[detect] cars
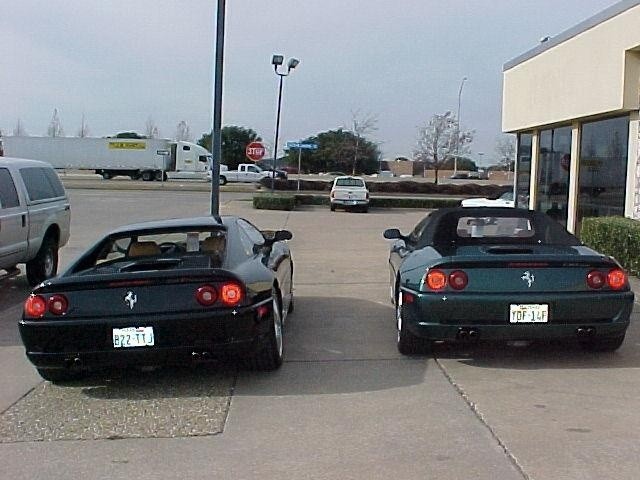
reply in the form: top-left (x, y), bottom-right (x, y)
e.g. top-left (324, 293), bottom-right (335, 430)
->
top-left (320, 170), bottom-right (490, 180)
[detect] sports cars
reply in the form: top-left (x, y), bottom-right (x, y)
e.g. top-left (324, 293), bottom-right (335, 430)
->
top-left (381, 206), bottom-right (635, 358)
top-left (16, 213), bottom-right (295, 387)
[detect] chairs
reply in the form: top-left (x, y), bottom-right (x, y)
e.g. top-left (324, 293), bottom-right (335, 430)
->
top-left (201, 237), bottom-right (227, 256)
top-left (128, 240), bottom-right (161, 257)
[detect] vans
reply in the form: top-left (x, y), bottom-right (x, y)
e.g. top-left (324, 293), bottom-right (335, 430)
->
top-left (0, 156), bottom-right (72, 289)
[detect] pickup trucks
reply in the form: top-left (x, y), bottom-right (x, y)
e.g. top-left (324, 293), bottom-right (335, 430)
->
top-left (218, 163), bottom-right (273, 185)
top-left (328, 175), bottom-right (371, 213)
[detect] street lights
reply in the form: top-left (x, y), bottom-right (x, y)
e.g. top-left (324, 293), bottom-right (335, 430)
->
top-left (270, 53), bottom-right (300, 193)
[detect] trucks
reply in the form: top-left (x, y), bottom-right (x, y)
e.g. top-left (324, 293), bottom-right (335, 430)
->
top-left (0, 135), bottom-right (213, 182)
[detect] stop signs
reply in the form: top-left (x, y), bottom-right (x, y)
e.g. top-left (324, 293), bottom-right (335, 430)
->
top-left (245, 141), bottom-right (266, 162)
